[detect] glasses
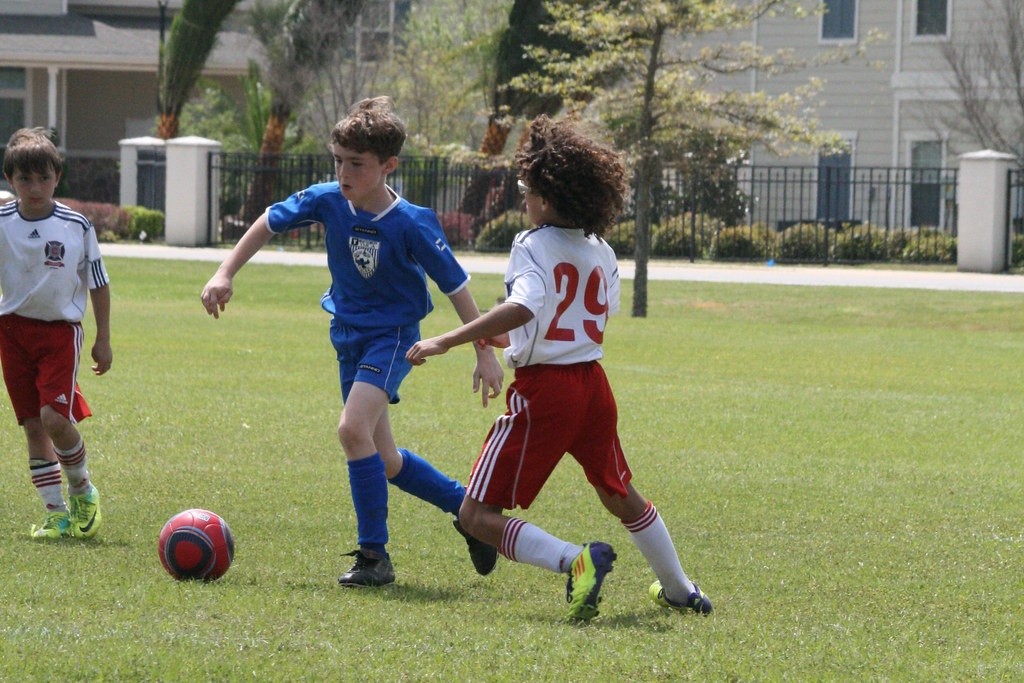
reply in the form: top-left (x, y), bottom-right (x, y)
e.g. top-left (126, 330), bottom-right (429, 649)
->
top-left (517, 180), bottom-right (537, 194)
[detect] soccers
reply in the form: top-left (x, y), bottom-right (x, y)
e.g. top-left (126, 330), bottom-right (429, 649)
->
top-left (158, 509), bottom-right (235, 583)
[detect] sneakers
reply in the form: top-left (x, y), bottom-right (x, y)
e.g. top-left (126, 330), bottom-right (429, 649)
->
top-left (566, 541), bottom-right (618, 625)
top-left (453, 487), bottom-right (503, 576)
top-left (649, 581), bottom-right (711, 614)
top-left (338, 550), bottom-right (396, 587)
top-left (33, 513), bottom-right (70, 539)
top-left (69, 484), bottom-right (101, 540)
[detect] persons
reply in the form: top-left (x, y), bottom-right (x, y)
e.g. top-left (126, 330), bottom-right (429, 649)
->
top-left (200, 94), bottom-right (506, 589)
top-left (405, 119), bottom-right (713, 625)
top-left (0, 126), bottom-right (113, 538)
